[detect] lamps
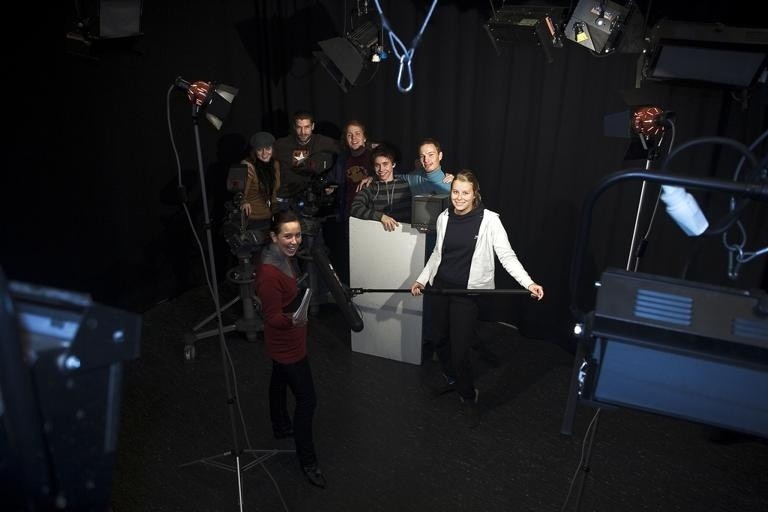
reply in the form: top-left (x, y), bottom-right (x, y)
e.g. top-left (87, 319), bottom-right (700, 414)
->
top-left (166, 75), bottom-right (310, 511)
top-left (563, 107), bottom-right (675, 510)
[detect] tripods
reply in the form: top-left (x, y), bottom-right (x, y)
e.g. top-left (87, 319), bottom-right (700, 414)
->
top-left (174, 114), bottom-right (298, 511)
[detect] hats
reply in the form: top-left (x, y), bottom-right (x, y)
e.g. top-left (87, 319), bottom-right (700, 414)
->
top-left (251, 132), bottom-right (275, 148)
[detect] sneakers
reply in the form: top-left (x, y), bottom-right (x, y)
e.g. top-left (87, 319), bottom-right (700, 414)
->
top-left (457, 389), bottom-right (480, 428)
top-left (275, 429), bottom-right (293, 438)
top-left (304, 469), bottom-right (328, 489)
top-left (418, 373), bottom-right (455, 397)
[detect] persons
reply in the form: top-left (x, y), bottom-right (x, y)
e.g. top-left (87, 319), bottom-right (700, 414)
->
top-left (223, 117), bottom-right (454, 341)
top-left (411, 170), bottom-right (543, 424)
top-left (256, 210), bottom-right (326, 487)
